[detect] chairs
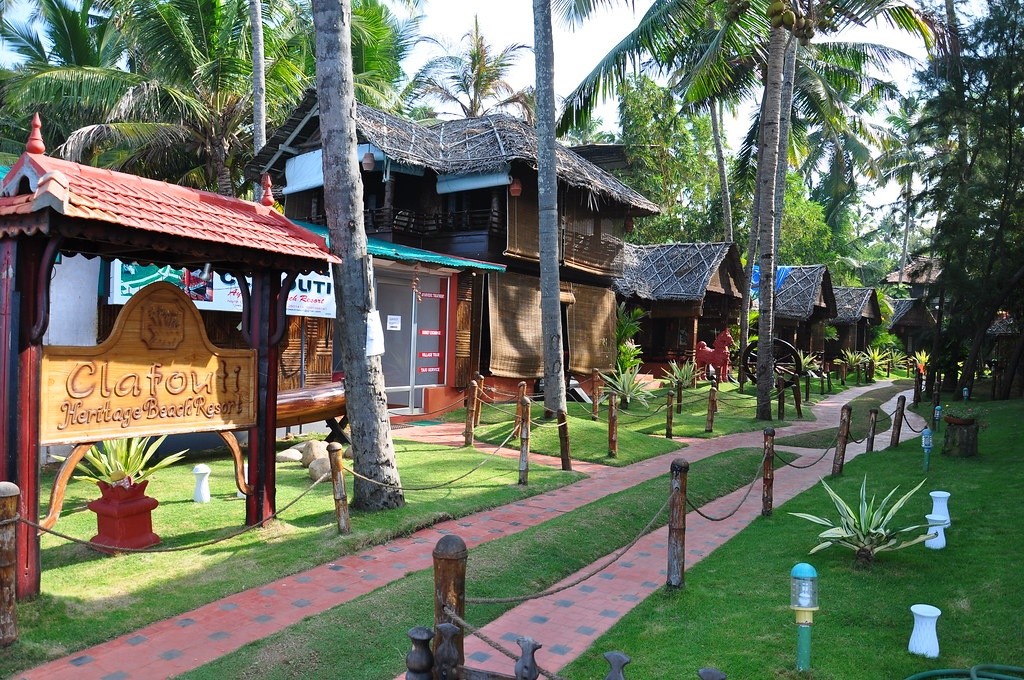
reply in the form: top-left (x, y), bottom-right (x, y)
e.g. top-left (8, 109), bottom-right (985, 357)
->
top-left (394, 210), bottom-right (415, 232)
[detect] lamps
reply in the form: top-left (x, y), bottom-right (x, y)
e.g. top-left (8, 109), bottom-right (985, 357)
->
top-left (791, 563), bottom-right (820, 611)
top-left (963, 388), bottom-right (969, 397)
top-left (934, 406), bottom-right (942, 418)
top-left (510, 176), bottom-right (522, 196)
top-left (921, 428), bottom-right (934, 448)
top-left (362, 140), bottom-right (375, 171)
top-left (624, 214), bottom-right (634, 232)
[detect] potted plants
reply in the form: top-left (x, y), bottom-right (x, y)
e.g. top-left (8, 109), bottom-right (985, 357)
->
top-left (942, 407), bottom-right (990, 425)
top-left (49, 434), bottom-right (190, 554)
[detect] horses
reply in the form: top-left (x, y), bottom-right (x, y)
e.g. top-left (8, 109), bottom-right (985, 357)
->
top-left (696, 327), bottom-right (734, 383)
